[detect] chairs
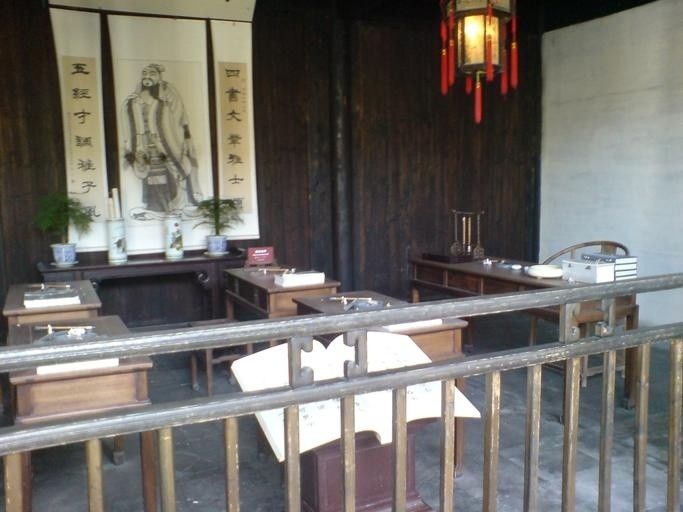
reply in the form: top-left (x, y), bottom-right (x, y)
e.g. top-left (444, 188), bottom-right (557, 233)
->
top-left (528, 241), bottom-right (632, 389)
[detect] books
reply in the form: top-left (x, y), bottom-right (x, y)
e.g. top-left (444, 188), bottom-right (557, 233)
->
top-left (273, 269), bottom-right (327, 289)
top-left (23, 289), bottom-right (80, 309)
top-left (582, 252), bottom-right (637, 281)
top-left (384, 319), bottom-right (443, 330)
top-left (37, 338), bottom-right (120, 376)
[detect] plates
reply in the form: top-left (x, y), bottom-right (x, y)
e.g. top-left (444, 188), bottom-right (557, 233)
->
top-left (527, 264), bottom-right (564, 278)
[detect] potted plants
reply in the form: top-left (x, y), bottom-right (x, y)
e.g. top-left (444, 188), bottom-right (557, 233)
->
top-left (195, 198), bottom-right (244, 256)
top-left (32, 194), bottom-right (95, 268)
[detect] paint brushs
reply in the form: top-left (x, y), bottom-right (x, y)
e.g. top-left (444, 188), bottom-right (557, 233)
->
top-left (29, 285), bottom-right (73, 288)
top-left (36, 325), bottom-right (96, 330)
top-left (328, 297), bottom-right (372, 300)
top-left (258, 268), bottom-right (297, 271)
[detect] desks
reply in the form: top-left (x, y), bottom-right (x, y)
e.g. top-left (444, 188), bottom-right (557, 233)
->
top-left (225, 267), bottom-right (342, 351)
top-left (7, 315), bottom-right (156, 511)
top-left (3, 281), bottom-right (102, 330)
top-left (292, 289), bottom-right (469, 479)
top-left (409, 257), bottom-right (640, 409)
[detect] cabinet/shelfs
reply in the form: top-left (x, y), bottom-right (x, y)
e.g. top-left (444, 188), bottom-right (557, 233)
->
top-left (36, 248), bottom-right (248, 320)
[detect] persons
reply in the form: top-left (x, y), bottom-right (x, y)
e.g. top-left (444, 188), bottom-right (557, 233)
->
top-left (120, 64), bottom-right (205, 218)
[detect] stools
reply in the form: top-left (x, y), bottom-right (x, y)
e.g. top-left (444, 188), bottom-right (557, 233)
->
top-left (190, 317), bottom-right (255, 399)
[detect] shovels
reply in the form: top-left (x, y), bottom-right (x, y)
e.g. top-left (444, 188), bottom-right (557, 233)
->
top-left (473, 214), bottom-right (484, 259)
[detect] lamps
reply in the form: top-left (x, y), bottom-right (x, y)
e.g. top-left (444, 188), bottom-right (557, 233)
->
top-left (440, 1), bottom-right (519, 126)
